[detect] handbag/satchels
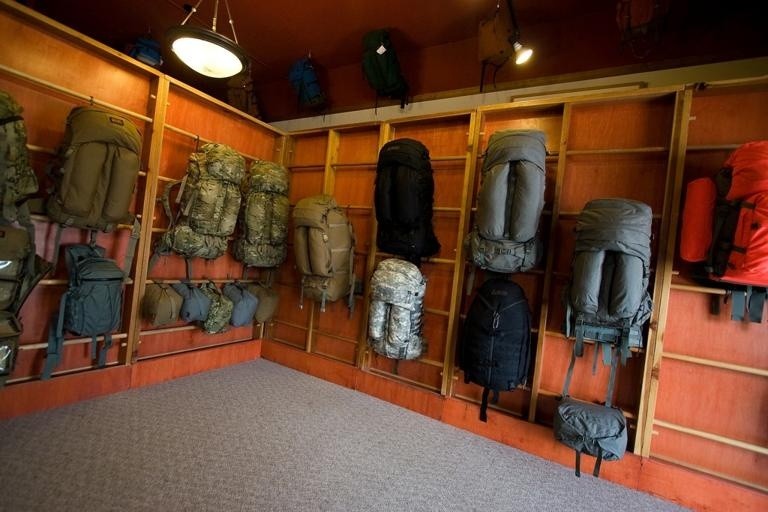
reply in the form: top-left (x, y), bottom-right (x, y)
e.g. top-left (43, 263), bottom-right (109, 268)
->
top-left (140, 280), bottom-right (281, 335)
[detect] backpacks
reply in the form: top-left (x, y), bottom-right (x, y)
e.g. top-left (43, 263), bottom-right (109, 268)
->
top-left (550, 396), bottom-right (629, 462)
top-left (231, 160), bottom-right (292, 270)
top-left (454, 276), bottom-right (529, 392)
top-left (560, 197), bottom-right (656, 350)
top-left (56, 244), bottom-right (122, 339)
top-left (221, 67), bottom-right (261, 116)
top-left (469, 127), bottom-right (548, 272)
top-left (291, 193), bottom-right (352, 303)
top-left (0, 226), bottom-right (46, 377)
top-left (165, 141), bottom-right (240, 258)
top-left (361, 28), bottom-right (411, 108)
top-left (374, 138), bottom-right (442, 256)
top-left (677, 138), bottom-right (768, 292)
top-left (42, 104), bottom-right (140, 233)
top-left (363, 256), bottom-right (428, 361)
top-left (289, 53), bottom-right (330, 113)
top-left (131, 39), bottom-right (169, 72)
top-left (0, 90), bottom-right (42, 222)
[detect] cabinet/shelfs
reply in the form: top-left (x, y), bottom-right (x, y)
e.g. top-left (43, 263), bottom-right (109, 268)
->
top-left (0, 0), bottom-right (768, 512)
